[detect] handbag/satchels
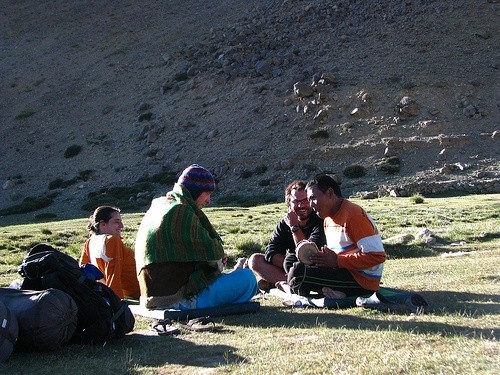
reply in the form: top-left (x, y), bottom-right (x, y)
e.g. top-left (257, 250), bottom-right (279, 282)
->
top-left (0, 285), bottom-right (85, 348)
top-left (0, 300), bottom-right (19, 362)
top-left (8, 243), bottom-right (135, 343)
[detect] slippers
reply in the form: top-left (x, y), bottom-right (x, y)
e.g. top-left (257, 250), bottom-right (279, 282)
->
top-left (180, 314), bottom-right (224, 332)
top-left (148, 318), bottom-right (181, 336)
top-left (405, 293), bottom-right (429, 314)
top-left (355, 292), bottom-right (399, 312)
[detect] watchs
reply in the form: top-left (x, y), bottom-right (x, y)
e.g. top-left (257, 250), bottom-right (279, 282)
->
top-left (291, 226), bottom-right (298, 232)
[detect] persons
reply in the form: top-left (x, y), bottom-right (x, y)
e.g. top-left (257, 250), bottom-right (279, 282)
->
top-left (134, 164), bottom-right (257, 309)
top-left (287, 175), bottom-right (386, 298)
top-left (79, 206), bottom-right (141, 299)
top-left (248, 181), bottom-right (327, 293)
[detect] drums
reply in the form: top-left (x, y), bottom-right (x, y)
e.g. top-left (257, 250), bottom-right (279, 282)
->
top-left (295, 240), bottom-right (318, 266)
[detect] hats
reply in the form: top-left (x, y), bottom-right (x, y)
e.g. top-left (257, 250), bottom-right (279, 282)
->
top-left (177, 164), bottom-right (216, 191)
